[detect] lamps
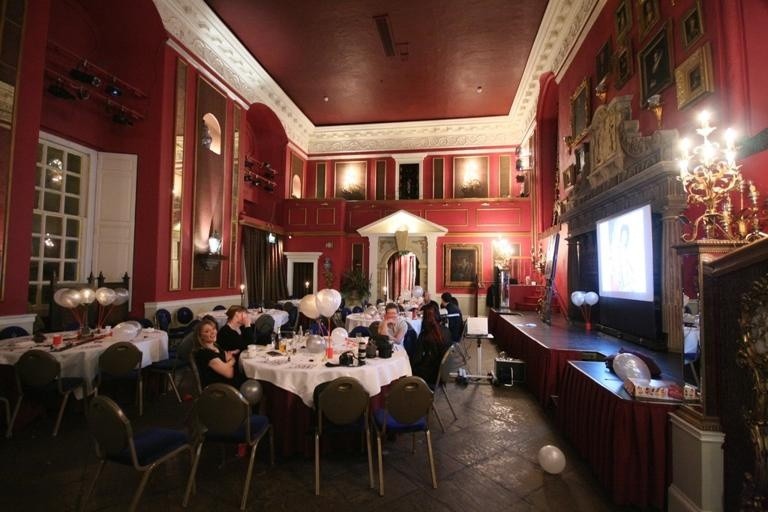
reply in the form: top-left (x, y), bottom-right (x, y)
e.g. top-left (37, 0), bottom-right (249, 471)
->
top-left (676, 105), bottom-right (763, 242)
top-left (208, 230), bottom-right (223, 254)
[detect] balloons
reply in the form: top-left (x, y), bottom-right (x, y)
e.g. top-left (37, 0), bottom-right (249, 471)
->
top-left (297, 288), bottom-right (343, 321)
top-left (111, 321), bottom-right (141, 342)
top-left (52, 287), bottom-right (128, 309)
top-left (535, 444), bottom-right (565, 474)
top-left (570, 290), bottom-right (598, 308)
top-left (610, 352), bottom-right (652, 387)
top-left (239, 379), bottom-right (262, 408)
top-left (411, 285), bottom-right (422, 299)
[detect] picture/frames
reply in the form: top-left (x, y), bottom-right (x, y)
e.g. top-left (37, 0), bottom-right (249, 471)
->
top-left (444, 243), bottom-right (482, 288)
top-left (491, 241), bottom-right (521, 281)
top-left (545, 0), bottom-right (713, 192)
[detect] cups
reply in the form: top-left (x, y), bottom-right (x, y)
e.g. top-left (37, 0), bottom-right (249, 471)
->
top-left (105, 325), bottom-right (113, 333)
top-left (247, 344), bottom-right (258, 360)
top-left (52, 335), bottom-right (62, 346)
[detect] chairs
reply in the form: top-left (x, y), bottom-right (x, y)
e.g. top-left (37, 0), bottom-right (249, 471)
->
top-left (182, 386), bottom-right (272, 512)
top-left (432, 345), bottom-right (457, 434)
top-left (314, 377), bottom-right (375, 495)
top-left (84, 397), bottom-right (196, 510)
top-left (4, 349), bottom-right (81, 435)
top-left (370, 376), bottom-right (437, 496)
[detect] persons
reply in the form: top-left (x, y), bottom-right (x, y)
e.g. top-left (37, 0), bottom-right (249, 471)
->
top-left (216, 305), bottom-right (252, 358)
top-left (193, 320), bottom-right (235, 388)
top-left (439, 292), bottom-right (463, 343)
top-left (377, 302), bottom-right (408, 346)
top-left (416, 290), bottom-right (438, 312)
top-left (412, 305), bottom-right (451, 390)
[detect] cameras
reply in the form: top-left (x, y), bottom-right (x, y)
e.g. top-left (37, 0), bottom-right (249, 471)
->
top-left (339, 355), bottom-right (352, 366)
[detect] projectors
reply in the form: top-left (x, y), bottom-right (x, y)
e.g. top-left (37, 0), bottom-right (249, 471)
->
top-left (467, 317), bottom-right (488, 335)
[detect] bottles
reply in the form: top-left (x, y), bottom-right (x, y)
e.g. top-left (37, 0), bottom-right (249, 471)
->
top-left (363, 296), bottom-right (417, 321)
top-left (258, 302), bottom-right (264, 313)
top-left (271, 324), bottom-right (363, 361)
